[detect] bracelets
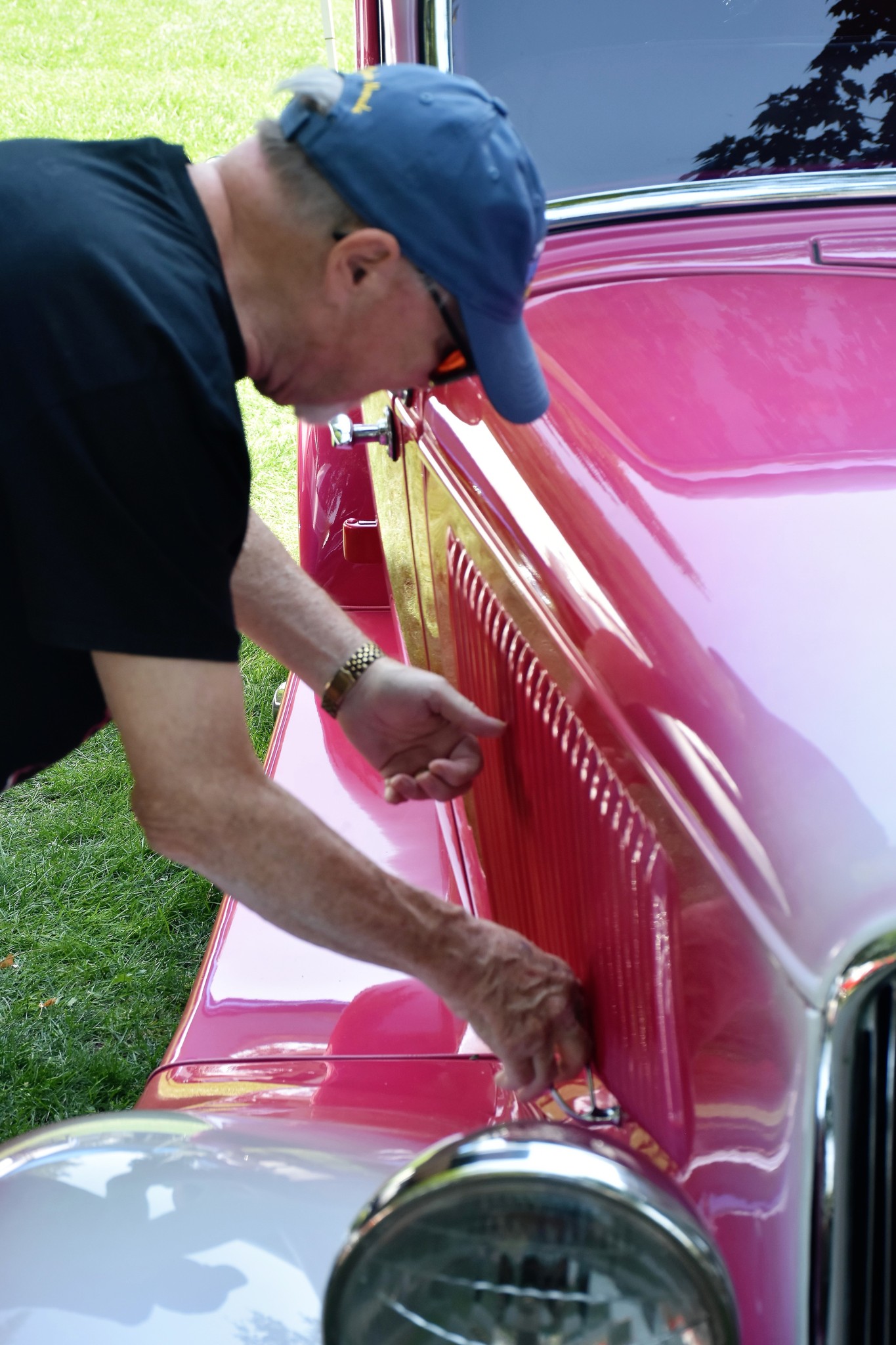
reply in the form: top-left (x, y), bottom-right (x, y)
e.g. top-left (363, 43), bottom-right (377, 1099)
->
top-left (319, 640), bottom-right (387, 717)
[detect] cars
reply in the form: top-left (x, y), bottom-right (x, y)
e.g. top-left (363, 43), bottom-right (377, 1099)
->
top-left (1, 1), bottom-right (896, 1344)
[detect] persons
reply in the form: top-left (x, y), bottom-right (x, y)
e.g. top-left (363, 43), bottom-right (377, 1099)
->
top-left (0, 62), bottom-right (593, 1103)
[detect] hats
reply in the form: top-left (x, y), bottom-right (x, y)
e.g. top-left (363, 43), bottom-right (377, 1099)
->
top-left (277, 62), bottom-right (553, 426)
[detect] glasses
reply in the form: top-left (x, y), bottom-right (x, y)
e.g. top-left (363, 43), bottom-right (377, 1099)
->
top-left (333, 226), bottom-right (481, 385)
